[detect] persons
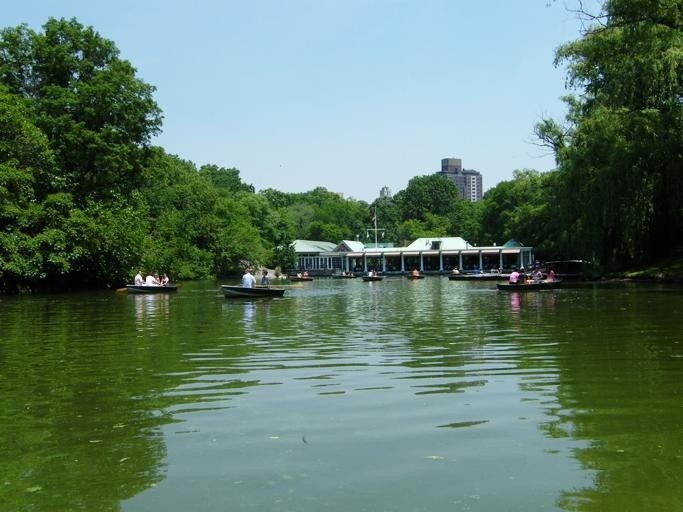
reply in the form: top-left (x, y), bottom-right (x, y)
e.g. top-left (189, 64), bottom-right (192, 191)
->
top-left (258, 269), bottom-right (270, 288)
top-left (508, 265), bottom-right (555, 285)
top-left (134, 270), bottom-right (143, 285)
top-left (145, 273), bottom-right (160, 286)
top-left (151, 271), bottom-right (160, 284)
top-left (159, 272), bottom-right (169, 286)
top-left (241, 268), bottom-right (255, 288)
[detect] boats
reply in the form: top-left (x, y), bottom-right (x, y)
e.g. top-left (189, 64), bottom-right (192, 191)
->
top-left (448, 272), bottom-right (511, 281)
top-left (333, 274), bottom-right (355, 279)
top-left (407, 275), bottom-right (425, 280)
top-left (495, 278), bottom-right (564, 292)
top-left (221, 284), bottom-right (285, 299)
top-left (362, 275), bottom-right (384, 281)
top-left (290, 275), bottom-right (313, 283)
top-left (126, 284), bottom-right (178, 295)
